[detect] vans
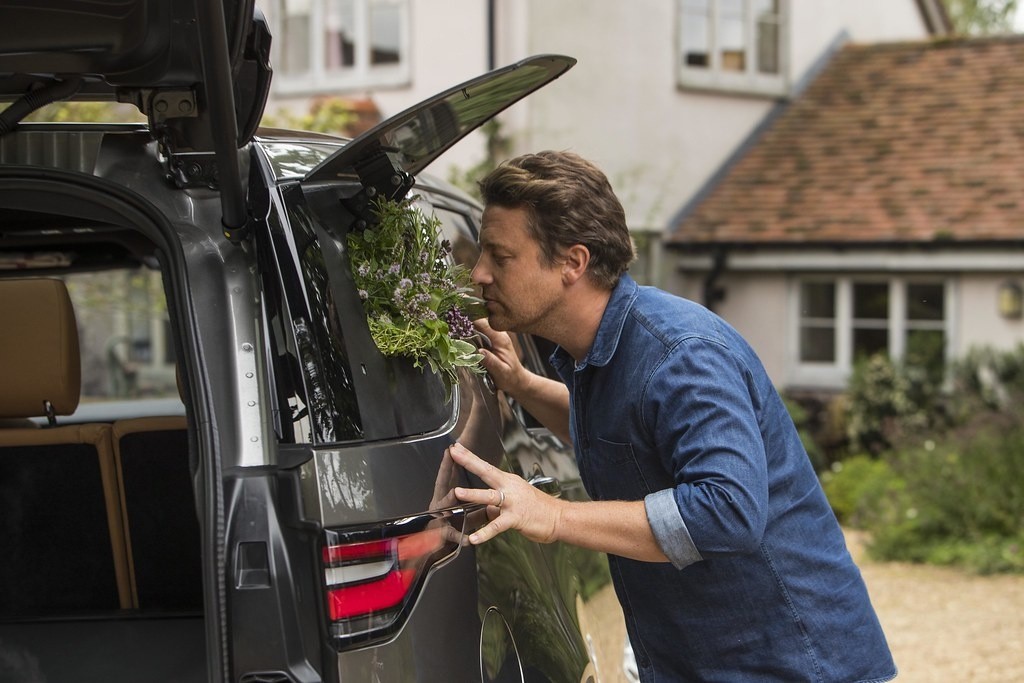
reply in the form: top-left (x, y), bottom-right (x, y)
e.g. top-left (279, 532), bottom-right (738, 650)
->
top-left (1, 0), bottom-right (578, 683)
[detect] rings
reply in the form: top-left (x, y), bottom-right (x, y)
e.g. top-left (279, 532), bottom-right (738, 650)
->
top-left (495, 490), bottom-right (505, 507)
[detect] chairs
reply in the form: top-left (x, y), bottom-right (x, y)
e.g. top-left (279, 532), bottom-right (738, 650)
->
top-left (0, 275), bottom-right (246, 625)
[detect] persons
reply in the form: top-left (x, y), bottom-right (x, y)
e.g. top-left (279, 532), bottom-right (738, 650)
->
top-left (388, 361), bottom-right (503, 570)
top-left (448, 150), bottom-right (897, 683)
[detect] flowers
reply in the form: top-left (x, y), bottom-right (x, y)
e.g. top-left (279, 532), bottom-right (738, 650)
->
top-left (342, 190), bottom-right (490, 402)
